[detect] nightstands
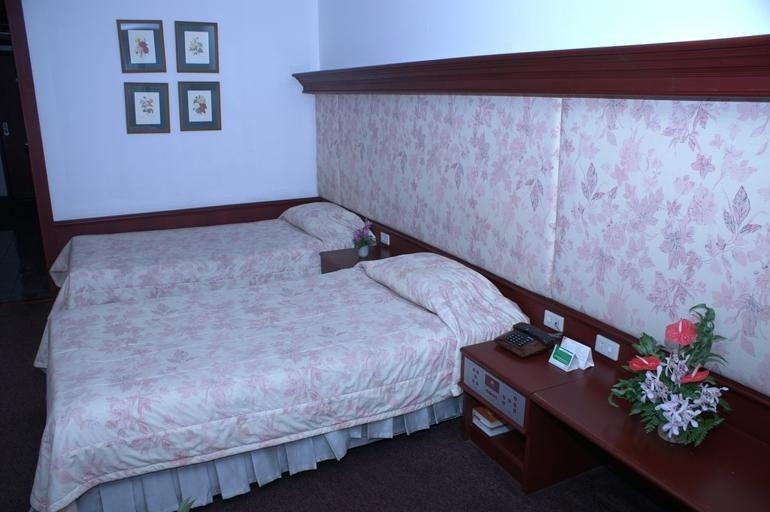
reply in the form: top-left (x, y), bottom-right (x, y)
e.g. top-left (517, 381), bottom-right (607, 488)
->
top-left (456, 324), bottom-right (621, 492)
top-left (319, 243), bottom-right (397, 273)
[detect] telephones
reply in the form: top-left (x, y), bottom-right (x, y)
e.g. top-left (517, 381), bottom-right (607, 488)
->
top-left (494, 322), bottom-right (554, 358)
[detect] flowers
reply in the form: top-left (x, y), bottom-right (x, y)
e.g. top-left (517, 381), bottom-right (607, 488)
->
top-left (614, 300), bottom-right (730, 443)
top-left (349, 216), bottom-right (379, 247)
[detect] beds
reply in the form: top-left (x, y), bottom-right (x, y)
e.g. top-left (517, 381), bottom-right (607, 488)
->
top-left (28, 245), bottom-right (532, 511)
top-left (34, 204), bottom-right (368, 365)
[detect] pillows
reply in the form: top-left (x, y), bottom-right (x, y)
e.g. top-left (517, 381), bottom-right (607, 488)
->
top-left (358, 251), bottom-right (494, 310)
top-left (279, 197), bottom-right (363, 247)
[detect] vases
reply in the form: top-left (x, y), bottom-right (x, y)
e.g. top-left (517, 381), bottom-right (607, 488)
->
top-left (657, 422), bottom-right (688, 442)
top-left (359, 244), bottom-right (368, 257)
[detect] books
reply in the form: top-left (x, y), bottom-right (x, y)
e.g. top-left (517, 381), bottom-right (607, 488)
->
top-left (472, 406), bottom-right (514, 440)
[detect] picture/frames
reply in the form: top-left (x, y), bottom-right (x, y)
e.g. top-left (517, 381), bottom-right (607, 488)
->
top-left (116, 19), bottom-right (221, 135)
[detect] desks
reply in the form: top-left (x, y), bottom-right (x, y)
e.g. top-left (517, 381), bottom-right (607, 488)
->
top-left (530, 348), bottom-right (770, 512)
